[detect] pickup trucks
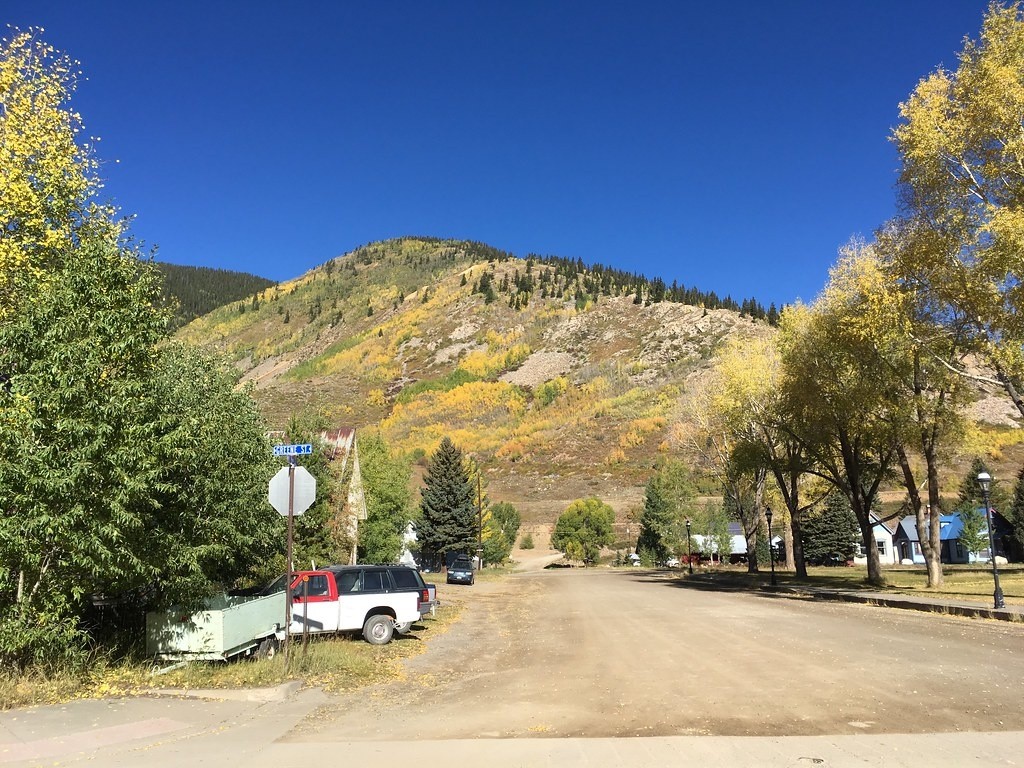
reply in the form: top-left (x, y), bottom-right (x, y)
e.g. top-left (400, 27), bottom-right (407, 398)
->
top-left (252, 571), bottom-right (423, 646)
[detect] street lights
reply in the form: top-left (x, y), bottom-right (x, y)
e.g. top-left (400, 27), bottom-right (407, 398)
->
top-left (976, 467), bottom-right (1005, 608)
top-left (765, 507), bottom-right (777, 586)
top-left (626, 527), bottom-right (631, 554)
top-left (685, 519), bottom-right (693, 575)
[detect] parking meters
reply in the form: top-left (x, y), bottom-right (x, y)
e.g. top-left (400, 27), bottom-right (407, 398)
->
top-left (302, 575), bottom-right (309, 654)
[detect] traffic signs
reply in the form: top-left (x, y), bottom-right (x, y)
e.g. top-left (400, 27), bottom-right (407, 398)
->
top-left (273, 444), bottom-right (313, 455)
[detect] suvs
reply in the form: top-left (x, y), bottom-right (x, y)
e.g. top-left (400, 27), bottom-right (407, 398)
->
top-left (447, 554), bottom-right (476, 585)
top-left (316, 564), bottom-right (430, 634)
